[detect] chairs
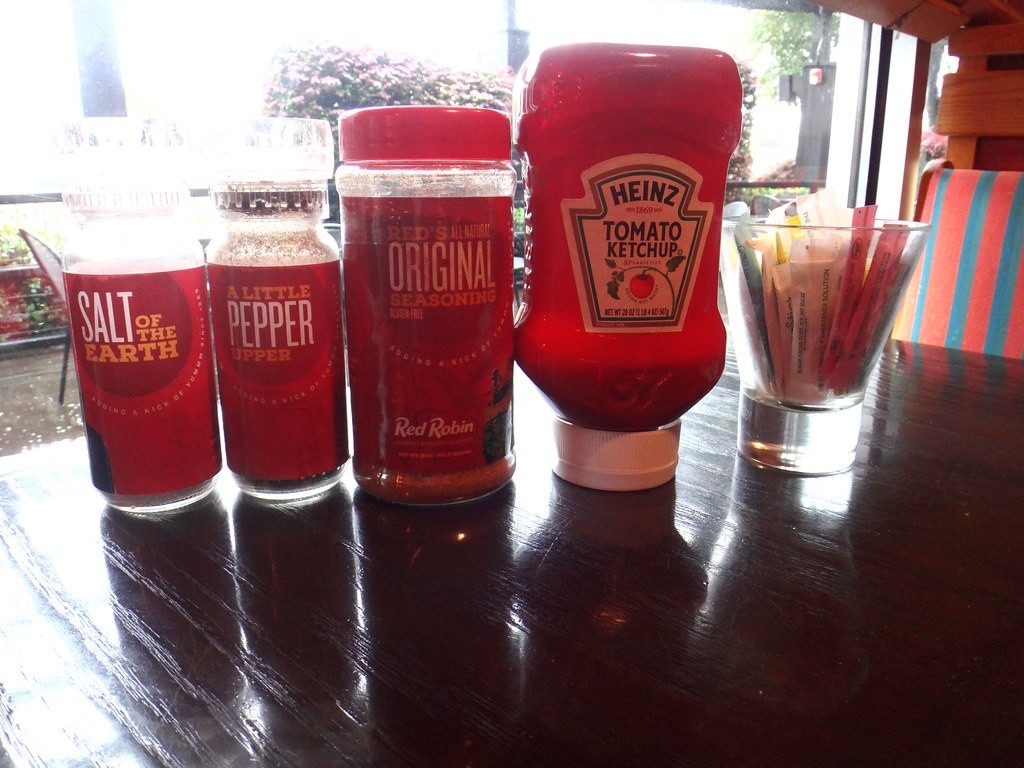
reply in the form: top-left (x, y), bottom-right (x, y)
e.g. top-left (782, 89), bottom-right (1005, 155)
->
top-left (886, 158), bottom-right (1024, 362)
top-left (19, 225), bottom-right (78, 407)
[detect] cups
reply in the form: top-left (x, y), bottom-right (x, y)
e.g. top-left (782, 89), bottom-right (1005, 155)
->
top-left (720, 219), bottom-right (933, 477)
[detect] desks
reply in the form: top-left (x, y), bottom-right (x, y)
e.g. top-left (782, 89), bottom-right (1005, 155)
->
top-left (1, 338), bottom-right (1023, 767)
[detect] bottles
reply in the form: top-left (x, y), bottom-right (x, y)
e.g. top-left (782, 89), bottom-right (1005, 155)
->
top-left (206, 118), bottom-right (351, 509)
top-left (59, 114), bottom-right (222, 519)
top-left (331, 105), bottom-right (517, 507)
top-left (512, 41), bottom-right (744, 492)
